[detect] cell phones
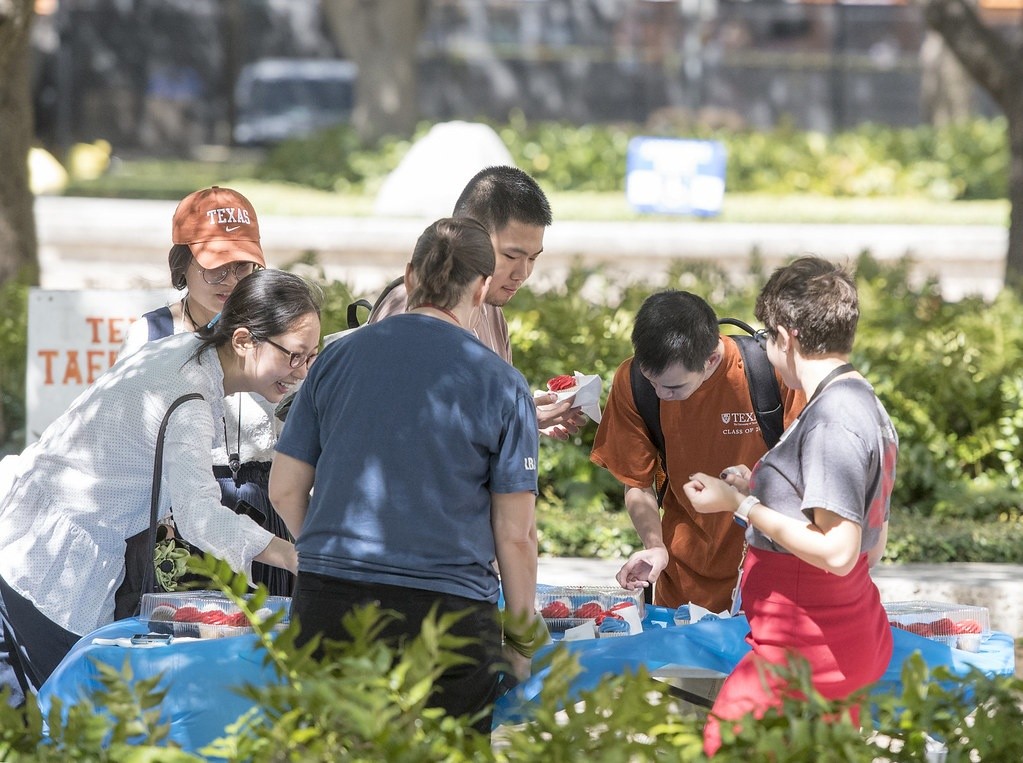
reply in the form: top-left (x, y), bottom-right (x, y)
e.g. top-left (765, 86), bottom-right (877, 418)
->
top-left (233, 500), bottom-right (267, 527)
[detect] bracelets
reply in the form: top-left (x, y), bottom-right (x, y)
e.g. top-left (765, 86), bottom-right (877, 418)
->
top-left (504, 632), bottom-right (536, 659)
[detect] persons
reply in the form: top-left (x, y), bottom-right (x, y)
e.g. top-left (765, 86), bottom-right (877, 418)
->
top-left (369, 165), bottom-right (553, 368)
top-left (682, 252), bottom-right (898, 760)
top-left (268, 217), bottom-right (539, 749)
top-left (587, 290), bottom-right (809, 616)
top-left (117, 186), bottom-right (310, 599)
top-left (0, 270), bottom-right (321, 720)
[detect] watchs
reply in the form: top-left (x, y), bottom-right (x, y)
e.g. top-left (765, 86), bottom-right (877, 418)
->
top-left (733, 496), bottom-right (760, 529)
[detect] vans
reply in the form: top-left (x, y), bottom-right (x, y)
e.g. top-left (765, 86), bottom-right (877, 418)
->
top-left (231, 56), bottom-right (359, 144)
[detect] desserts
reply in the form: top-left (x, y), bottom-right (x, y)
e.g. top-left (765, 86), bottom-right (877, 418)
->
top-left (547, 375), bottom-right (580, 398)
top-left (675, 604), bottom-right (727, 627)
top-left (889, 619), bottom-right (983, 638)
top-left (148, 603), bottom-right (271, 638)
top-left (534, 592), bottom-right (638, 638)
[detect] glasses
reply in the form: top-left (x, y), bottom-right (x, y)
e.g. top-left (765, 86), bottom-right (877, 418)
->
top-left (188, 262), bottom-right (258, 285)
top-left (260, 336), bottom-right (318, 370)
top-left (754, 324), bottom-right (798, 352)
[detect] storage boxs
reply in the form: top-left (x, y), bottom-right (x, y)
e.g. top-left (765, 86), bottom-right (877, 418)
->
top-left (139, 590), bottom-right (292, 641)
top-left (530, 584), bottom-right (649, 634)
top-left (881, 601), bottom-right (993, 650)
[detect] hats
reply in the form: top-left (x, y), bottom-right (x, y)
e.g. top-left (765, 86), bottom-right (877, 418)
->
top-left (172, 185), bottom-right (265, 269)
